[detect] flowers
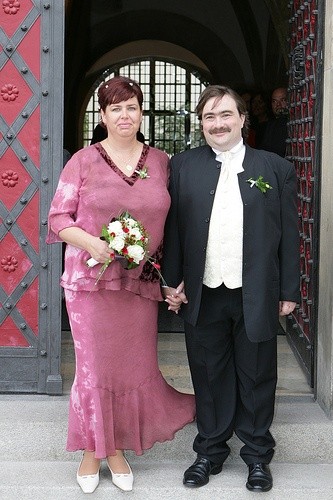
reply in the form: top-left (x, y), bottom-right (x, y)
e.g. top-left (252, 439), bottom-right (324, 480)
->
top-left (135, 166), bottom-right (151, 179)
top-left (246, 176), bottom-right (273, 193)
top-left (86, 208), bottom-right (168, 300)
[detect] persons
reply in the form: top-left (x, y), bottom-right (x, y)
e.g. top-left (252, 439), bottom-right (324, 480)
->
top-left (238, 86), bottom-right (290, 157)
top-left (90, 121), bottom-right (146, 146)
top-left (162, 84), bottom-right (302, 492)
top-left (44, 76), bottom-right (195, 494)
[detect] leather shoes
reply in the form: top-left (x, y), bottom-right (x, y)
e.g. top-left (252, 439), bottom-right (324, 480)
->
top-left (76, 453), bottom-right (100, 494)
top-left (183, 456), bottom-right (223, 487)
top-left (246, 463), bottom-right (273, 492)
top-left (106, 455), bottom-right (134, 491)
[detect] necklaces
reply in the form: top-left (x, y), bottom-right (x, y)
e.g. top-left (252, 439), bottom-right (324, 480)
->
top-left (104, 139), bottom-right (141, 173)
